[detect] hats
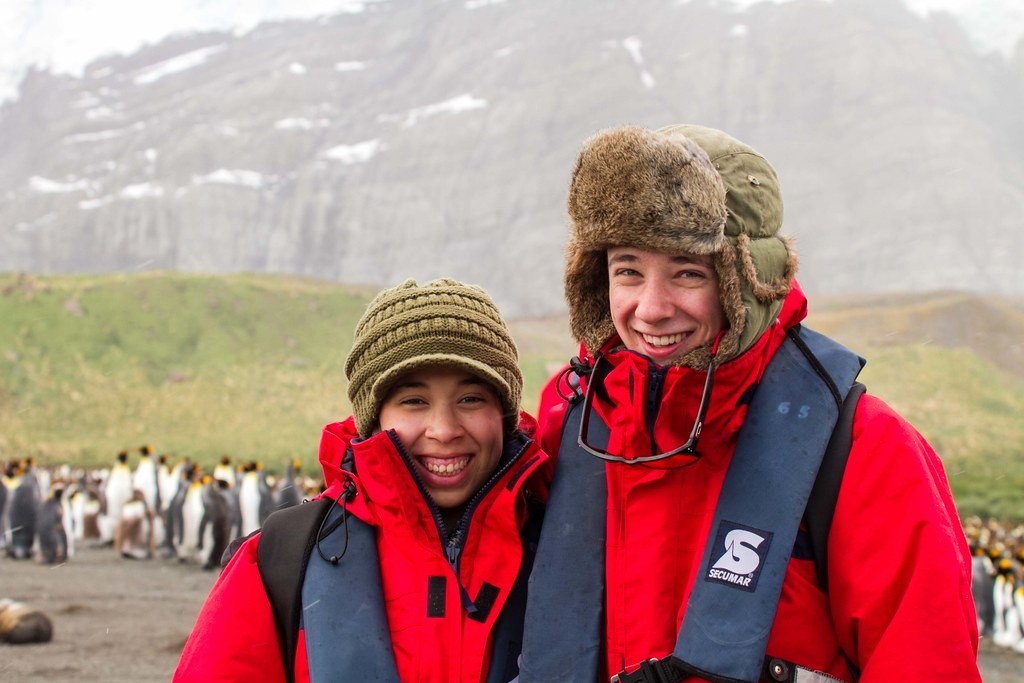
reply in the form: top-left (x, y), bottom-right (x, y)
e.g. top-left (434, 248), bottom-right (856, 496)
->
top-left (341, 280), bottom-right (525, 442)
top-left (564, 126), bottom-right (796, 369)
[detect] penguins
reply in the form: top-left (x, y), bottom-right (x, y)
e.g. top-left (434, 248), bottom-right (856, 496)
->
top-left (961, 513), bottom-right (1024, 657)
top-left (1, 442), bottom-right (326, 574)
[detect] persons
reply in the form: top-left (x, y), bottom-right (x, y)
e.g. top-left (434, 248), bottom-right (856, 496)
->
top-left (173, 277), bottom-right (549, 683)
top-left (518, 124), bottom-right (985, 683)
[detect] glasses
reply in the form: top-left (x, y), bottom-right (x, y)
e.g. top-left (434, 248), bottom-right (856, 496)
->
top-left (575, 346), bottom-right (715, 468)
